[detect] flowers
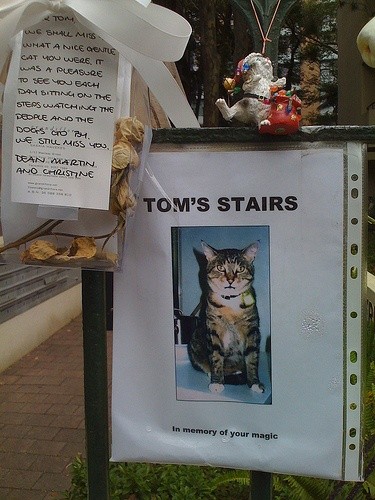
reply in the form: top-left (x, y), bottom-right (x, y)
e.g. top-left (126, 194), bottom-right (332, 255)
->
top-left (0, 115), bottom-right (145, 265)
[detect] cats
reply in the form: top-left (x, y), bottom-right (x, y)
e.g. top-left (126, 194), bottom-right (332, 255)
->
top-left (187, 238), bottom-right (266, 394)
